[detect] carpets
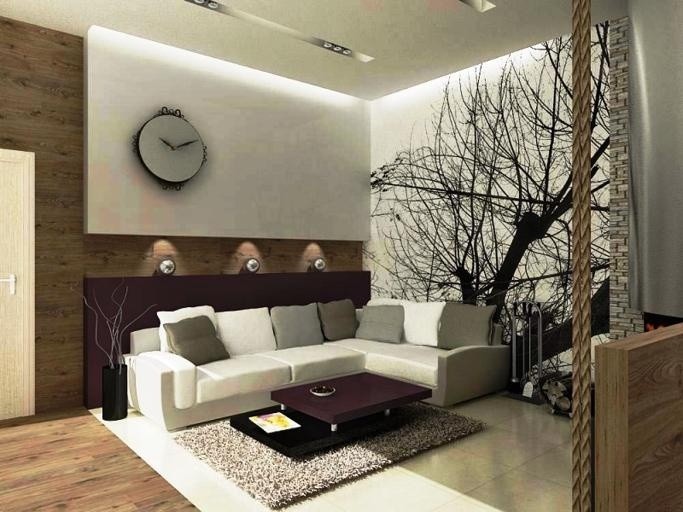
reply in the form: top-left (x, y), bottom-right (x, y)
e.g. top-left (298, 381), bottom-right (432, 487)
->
top-left (171, 403), bottom-right (485, 512)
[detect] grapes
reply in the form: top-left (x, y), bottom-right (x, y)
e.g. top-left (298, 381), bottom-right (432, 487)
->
top-left (310, 383), bottom-right (334, 393)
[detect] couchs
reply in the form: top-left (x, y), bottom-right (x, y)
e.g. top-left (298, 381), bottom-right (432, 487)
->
top-left (126, 307), bottom-right (512, 434)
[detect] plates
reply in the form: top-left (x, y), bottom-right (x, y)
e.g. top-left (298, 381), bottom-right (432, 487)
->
top-left (309, 385), bottom-right (336, 397)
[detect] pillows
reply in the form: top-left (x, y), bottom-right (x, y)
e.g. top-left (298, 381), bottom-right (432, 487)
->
top-left (154, 298), bottom-right (359, 365)
top-left (353, 296), bottom-right (499, 351)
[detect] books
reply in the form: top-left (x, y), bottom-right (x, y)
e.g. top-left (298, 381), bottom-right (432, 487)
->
top-left (249, 412), bottom-right (303, 434)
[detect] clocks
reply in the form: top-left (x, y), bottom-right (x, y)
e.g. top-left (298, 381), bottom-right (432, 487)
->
top-left (132, 105), bottom-right (209, 191)
